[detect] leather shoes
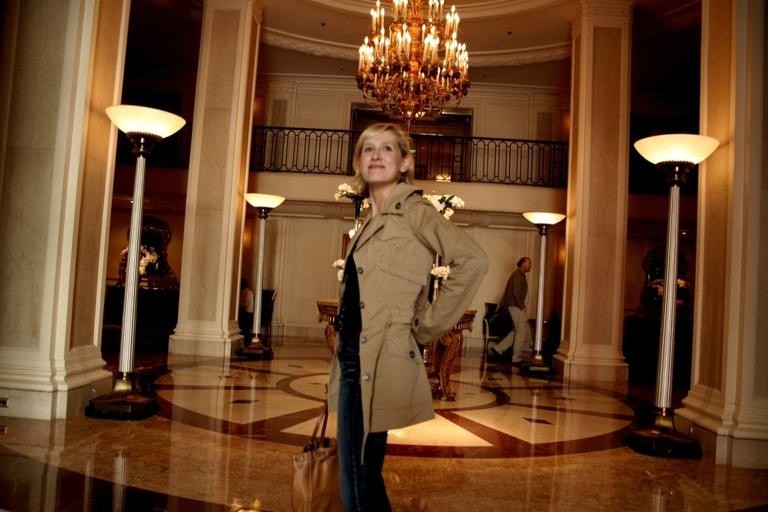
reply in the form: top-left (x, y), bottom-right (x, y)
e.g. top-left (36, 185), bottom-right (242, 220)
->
top-left (512, 361), bottom-right (530, 368)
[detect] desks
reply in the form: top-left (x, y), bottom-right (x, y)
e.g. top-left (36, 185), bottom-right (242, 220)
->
top-left (317, 300), bottom-right (477, 403)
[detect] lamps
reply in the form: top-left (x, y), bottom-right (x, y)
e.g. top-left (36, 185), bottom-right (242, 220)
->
top-left (354, 2), bottom-right (472, 137)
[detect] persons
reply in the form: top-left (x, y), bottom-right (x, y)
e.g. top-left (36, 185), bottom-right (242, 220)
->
top-left (327, 123), bottom-right (494, 512)
top-left (240, 277), bottom-right (254, 348)
top-left (492, 256), bottom-right (532, 367)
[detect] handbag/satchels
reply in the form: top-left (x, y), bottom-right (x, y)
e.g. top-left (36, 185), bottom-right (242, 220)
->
top-left (290, 437), bottom-right (344, 512)
